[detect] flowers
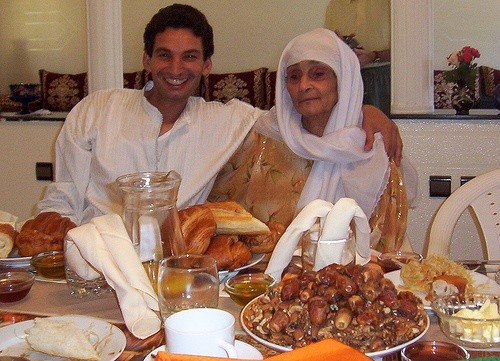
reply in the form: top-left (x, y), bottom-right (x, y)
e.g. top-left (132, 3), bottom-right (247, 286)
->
top-left (443, 46), bottom-right (480, 84)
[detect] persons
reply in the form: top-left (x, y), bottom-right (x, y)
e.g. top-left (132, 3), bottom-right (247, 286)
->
top-left (34, 3), bottom-right (405, 225)
top-left (206, 26), bottom-right (408, 254)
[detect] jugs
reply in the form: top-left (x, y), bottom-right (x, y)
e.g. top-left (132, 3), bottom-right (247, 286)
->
top-left (115, 171), bottom-right (193, 299)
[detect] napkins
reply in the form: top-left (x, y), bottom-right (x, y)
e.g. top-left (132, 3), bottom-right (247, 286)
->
top-left (314, 198), bottom-right (372, 271)
top-left (265, 199), bottom-right (333, 283)
top-left (64, 213), bottom-right (161, 338)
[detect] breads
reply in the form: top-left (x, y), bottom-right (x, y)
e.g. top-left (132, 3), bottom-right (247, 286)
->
top-left (159, 201), bottom-right (271, 270)
top-left (427, 275), bottom-right (467, 304)
top-left (0, 211), bottom-right (77, 259)
top-left (25, 317), bottom-right (99, 360)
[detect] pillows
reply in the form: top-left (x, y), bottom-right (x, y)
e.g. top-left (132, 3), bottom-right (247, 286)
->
top-left (263, 72), bottom-right (276, 111)
top-left (124, 69), bottom-right (144, 88)
top-left (38, 66), bottom-right (89, 111)
top-left (204, 65), bottom-right (268, 109)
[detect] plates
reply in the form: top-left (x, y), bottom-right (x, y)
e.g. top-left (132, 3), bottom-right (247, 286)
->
top-left (240, 294), bottom-right (430, 359)
top-left (143, 339), bottom-right (263, 361)
top-left (383, 269), bottom-right (500, 314)
top-left (0, 316), bottom-right (126, 361)
top-left (218, 251), bottom-right (266, 274)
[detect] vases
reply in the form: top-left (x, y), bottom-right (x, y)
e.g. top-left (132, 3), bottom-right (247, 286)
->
top-left (451, 82), bottom-right (474, 115)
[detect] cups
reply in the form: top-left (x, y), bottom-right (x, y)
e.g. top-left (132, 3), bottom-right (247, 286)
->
top-left (63, 237), bottom-right (115, 299)
top-left (400, 341), bottom-right (470, 361)
top-left (377, 251), bottom-right (424, 274)
top-left (164, 308), bottom-right (237, 359)
top-left (301, 229), bottom-right (357, 273)
top-left (158, 253), bottom-right (220, 322)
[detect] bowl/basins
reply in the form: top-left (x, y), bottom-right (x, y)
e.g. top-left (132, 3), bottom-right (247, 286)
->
top-left (224, 273), bottom-right (276, 305)
top-left (432, 294), bottom-right (500, 349)
top-left (0, 250), bottom-right (66, 303)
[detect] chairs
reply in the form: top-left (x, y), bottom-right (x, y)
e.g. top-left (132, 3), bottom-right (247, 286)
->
top-left (426, 169), bottom-right (500, 260)
